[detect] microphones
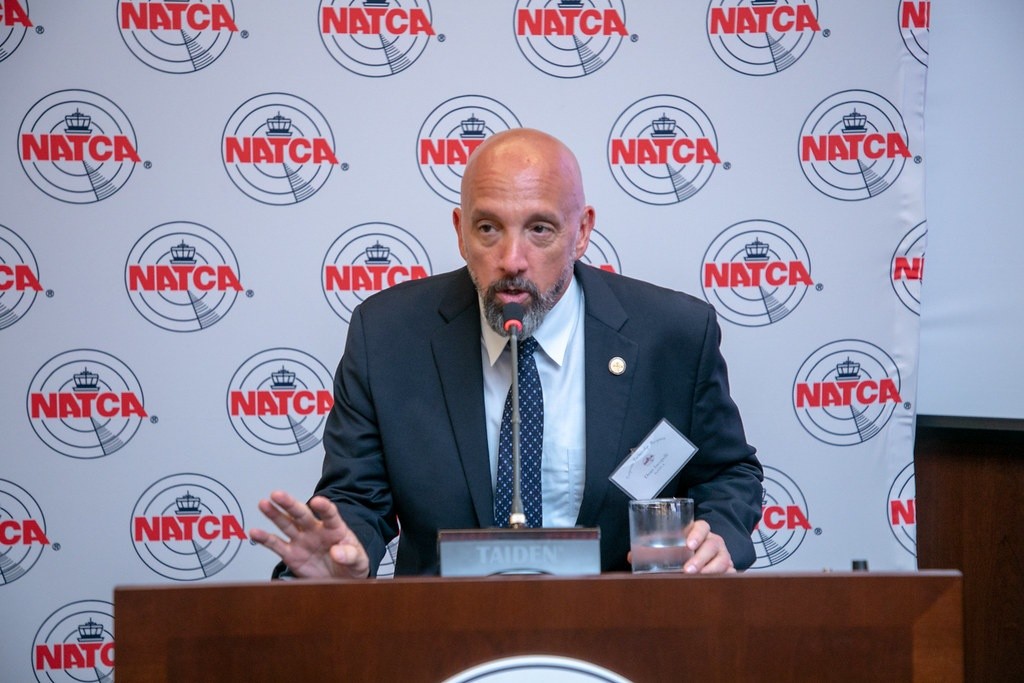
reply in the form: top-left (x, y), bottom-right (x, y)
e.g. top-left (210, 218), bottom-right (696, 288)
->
top-left (435, 301), bottom-right (603, 575)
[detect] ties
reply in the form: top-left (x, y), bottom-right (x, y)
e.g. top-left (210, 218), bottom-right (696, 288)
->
top-left (493, 337), bottom-right (544, 529)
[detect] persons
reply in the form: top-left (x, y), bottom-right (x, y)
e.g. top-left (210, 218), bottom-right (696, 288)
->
top-left (250, 128), bottom-right (765, 581)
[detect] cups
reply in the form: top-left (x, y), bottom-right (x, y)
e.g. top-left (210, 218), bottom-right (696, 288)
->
top-left (628, 498), bottom-right (695, 574)
